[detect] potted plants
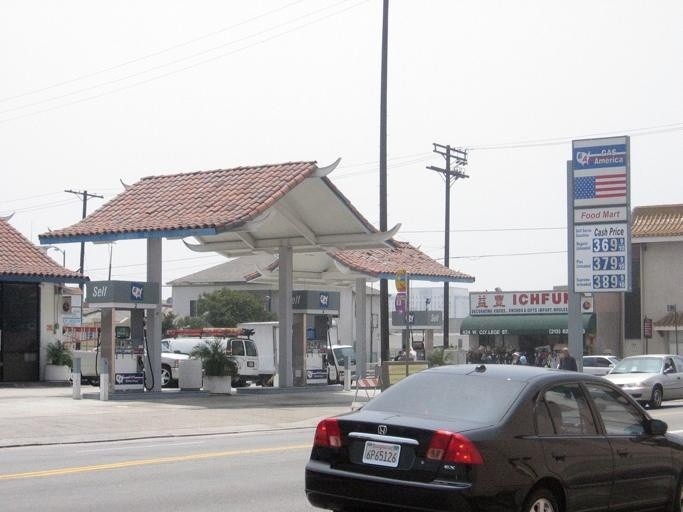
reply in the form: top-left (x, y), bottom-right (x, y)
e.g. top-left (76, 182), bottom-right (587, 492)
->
top-left (44, 341), bottom-right (73, 381)
top-left (188, 336), bottom-right (238, 395)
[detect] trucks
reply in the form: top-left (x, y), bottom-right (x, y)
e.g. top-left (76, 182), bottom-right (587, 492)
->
top-left (83, 322), bottom-right (358, 389)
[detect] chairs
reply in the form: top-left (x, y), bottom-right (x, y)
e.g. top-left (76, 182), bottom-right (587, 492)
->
top-left (534, 401), bottom-right (565, 436)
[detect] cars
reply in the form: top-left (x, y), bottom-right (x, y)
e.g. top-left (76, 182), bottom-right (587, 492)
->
top-left (557, 353), bottom-right (620, 376)
top-left (599, 354), bottom-right (683, 409)
top-left (303, 361), bottom-right (683, 512)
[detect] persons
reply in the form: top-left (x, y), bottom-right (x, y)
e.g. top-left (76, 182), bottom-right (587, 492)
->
top-left (469, 345), bottom-right (559, 369)
top-left (560, 347), bottom-right (577, 400)
